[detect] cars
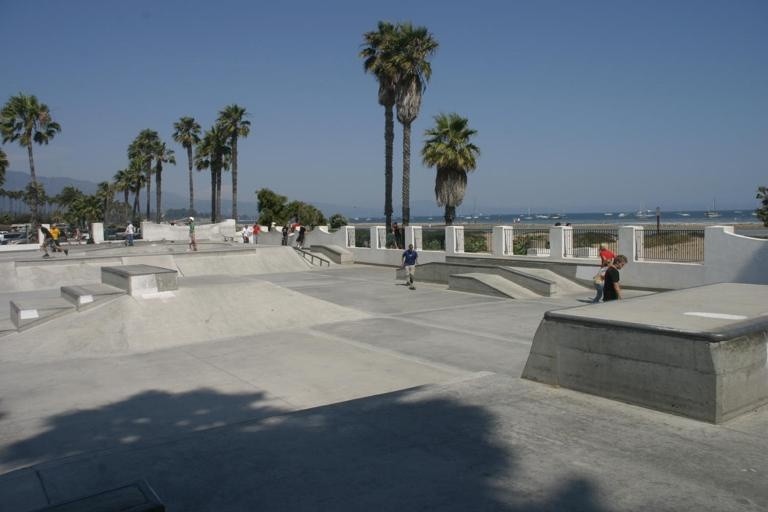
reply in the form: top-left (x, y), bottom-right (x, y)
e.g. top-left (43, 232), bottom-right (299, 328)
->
top-left (0, 222), bottom-right (143, 245)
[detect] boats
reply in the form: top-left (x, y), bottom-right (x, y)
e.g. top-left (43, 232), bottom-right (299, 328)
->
top-left (513, 212), bottom-right (562, 222)
top-left (675, 211), bottom-right (758, 218)
top-left (353, 217), bottom-right (373, 221)
top-left (410, 217), bottom-right (433, 221)
top-left (459, 213), bottom-right (491, 220)
top-left (603, 210), bottom-right (657, 219)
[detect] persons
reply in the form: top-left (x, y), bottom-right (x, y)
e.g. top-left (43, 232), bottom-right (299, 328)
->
top-left (296, 224), bottom-right (307, 247)
top-left (598, 245), bottom-right (616, 264)
top-left (35, 223), bottom-right (69, 258)
top-left (125, 222), bottom-right (135, 247)
top-left (601, 255), bottom-right (628, 302)
top-left (392, 222), bottom-right (401, 246)
top-left (241, 224), bottom-right (250, 243)
top-left (72, 227), bottom-right (81, 242)
top-left (281, 225), bottom-right (289, 245)
top-left (252, 222), bottom-right (261, 244)
top-left (589, 261), bottom-right (611, 304)
top-left (401, 244), bottom-right (419, 290)
top-left (187, 219), bottom-right (198, 251)
top-left (49, 223), bottom-right (62, 253)
